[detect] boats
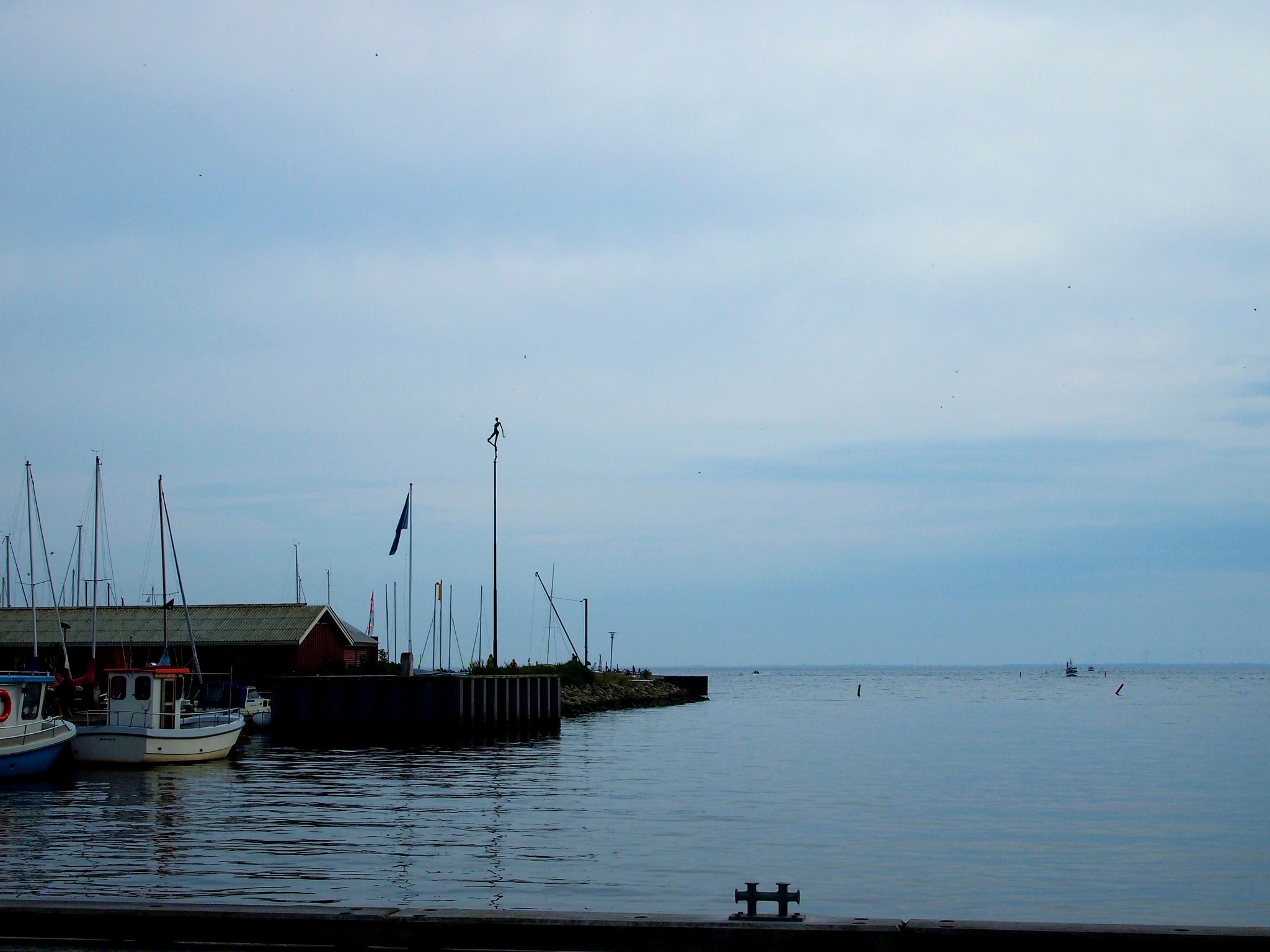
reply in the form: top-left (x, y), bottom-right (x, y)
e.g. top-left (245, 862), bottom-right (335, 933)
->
top-left (253, 712), bottom-right (272, 726)
top-left (1066, 660), bottom-right (1078, 677)
top-left (1088, 665), bottom-right (1095, 671)
top-left (231, 684), bottom-right (272, 726)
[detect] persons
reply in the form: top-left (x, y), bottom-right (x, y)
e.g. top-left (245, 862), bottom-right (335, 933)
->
top-left (632, 665), bottom-right (636, 674)
top-left (572, 653), bottom-right (577, 661)
top-left (509, 659), bottom-right (517, 668)
top-left (487, 654), bottom-right (494, 667)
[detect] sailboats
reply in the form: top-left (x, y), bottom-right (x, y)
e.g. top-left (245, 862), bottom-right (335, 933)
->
top-left (0, 444), bottom-right (245, 780)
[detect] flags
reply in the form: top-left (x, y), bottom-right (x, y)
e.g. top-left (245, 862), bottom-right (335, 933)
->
top-left (389, 491), bottom-right (408, 556)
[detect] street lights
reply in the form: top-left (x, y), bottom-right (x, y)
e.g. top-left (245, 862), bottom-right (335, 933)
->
top-left (608, 632), bottom-right (617, 671)
